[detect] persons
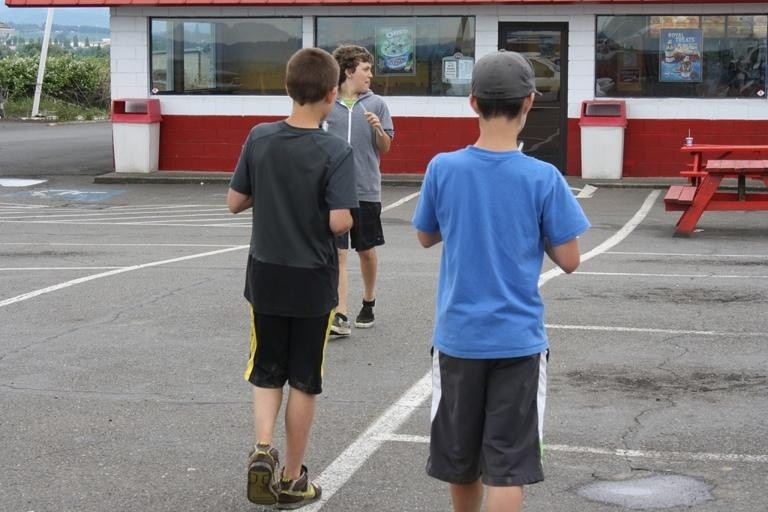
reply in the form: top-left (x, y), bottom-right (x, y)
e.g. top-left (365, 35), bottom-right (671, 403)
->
top-left (320, 45), bottom-right (394, 335)
top-left (228, 48), bottom-right (360, 510)
top-left (411, 48), bottom-right (593, 512)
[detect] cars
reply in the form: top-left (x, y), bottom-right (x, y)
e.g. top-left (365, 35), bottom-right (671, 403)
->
top-left (595, 76), bottom-right (616, 97)
top-left (519, 51), bottom-right (560, 112)
top-left (205, 71), bottom-right (240, 93)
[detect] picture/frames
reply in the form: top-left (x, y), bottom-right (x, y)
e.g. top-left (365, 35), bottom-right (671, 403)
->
top-left (658, 27), bottom-right (703, 83)
top-left (374, 23), bottom-right (416, 77)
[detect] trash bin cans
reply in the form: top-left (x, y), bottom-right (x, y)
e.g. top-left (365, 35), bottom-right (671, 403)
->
top-left (578, 100), bottom-right (628, 180)
top-left (111, 98), bottom-right (164, 173)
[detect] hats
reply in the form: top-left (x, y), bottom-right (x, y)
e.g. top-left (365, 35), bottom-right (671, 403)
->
top-left (472, 49), bottom-right (542, 99)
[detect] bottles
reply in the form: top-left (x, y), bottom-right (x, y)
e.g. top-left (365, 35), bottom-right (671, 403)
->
top-left (665, 40), bottom-right (675, 63)
top-left (681, 56), bottom-right (691, 78)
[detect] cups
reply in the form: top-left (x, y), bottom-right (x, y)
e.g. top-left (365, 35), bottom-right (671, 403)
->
top-left (685, 137), bottom-right (693, 147)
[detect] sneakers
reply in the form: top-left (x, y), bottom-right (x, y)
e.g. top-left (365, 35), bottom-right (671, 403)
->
top-left (330, 313), bottom-right (351, 336)
top-left (355, 298), bottom-right (376, 328)
top-left (246, 441), bottom-right (279, 505)
top-left (277, 464), bottom-right (321, 510)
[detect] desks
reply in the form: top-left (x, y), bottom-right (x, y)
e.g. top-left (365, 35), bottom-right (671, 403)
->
top-left (680, 144), bottom-right (767, 181)
top-left (674, 159), bottom-right (767, 236)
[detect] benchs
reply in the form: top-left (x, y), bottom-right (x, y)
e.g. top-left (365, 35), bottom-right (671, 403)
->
top-left (665, 163), bottom-right (767, 237)
